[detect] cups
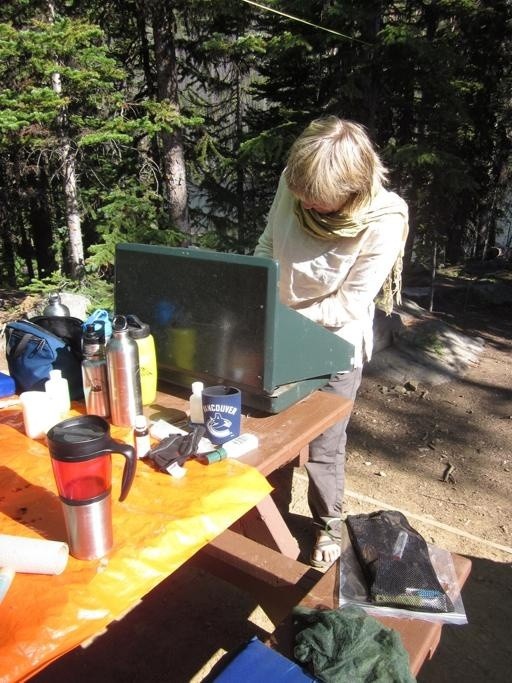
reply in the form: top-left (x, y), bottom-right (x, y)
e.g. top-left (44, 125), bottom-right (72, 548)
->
top-left (19, 391), bottom-right (47, 441)
top-left (201, 384), bottom-right (241, 445)
top-left (45, 411), bottom-right (137, 561)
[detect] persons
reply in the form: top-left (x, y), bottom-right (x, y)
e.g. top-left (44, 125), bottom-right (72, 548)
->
top-left (250, 118), bottom-right (413, 568)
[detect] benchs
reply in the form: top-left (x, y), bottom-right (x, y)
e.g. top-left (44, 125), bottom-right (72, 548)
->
top-left (264, 539), bottom-right (475, 683)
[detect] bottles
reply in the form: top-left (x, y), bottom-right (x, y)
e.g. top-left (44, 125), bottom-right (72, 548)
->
top-left (81, 308), bottom-right (114, 351)
top-left (43, 289), bottom-right (71, 317)
top-left (134, 414), bottom-right (150, 459)
top-left (189, 382), bottom-right (204, 425)
top-left (44, 369), bottom-right (72, 414)
top-left (128, 314), bottom-right (158, 405)
top-left (206, 433), bottom-right (258, 464)
top-left (107, 315), bottom-right (143, 427)
top-left (81, 318), bottom-right (112, 418)
top-left (171, 306), bottom-right (198, 370)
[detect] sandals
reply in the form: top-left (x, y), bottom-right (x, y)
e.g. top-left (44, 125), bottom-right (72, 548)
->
top-left (309, 516), bottom-right (341, 569)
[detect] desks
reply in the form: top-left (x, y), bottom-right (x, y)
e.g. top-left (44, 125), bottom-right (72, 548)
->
top-left (1, 388), bottom-right (355, 683)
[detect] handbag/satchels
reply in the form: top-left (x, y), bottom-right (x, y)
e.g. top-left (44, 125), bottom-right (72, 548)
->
top-left (7, 315), bottom-right (83, 401)
